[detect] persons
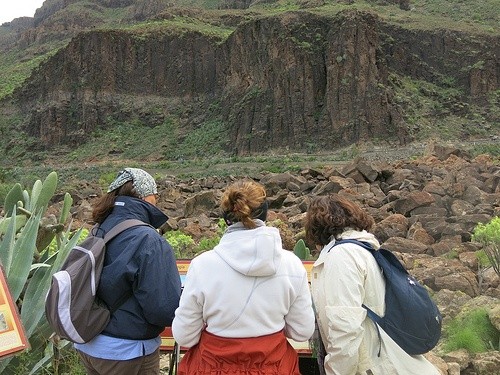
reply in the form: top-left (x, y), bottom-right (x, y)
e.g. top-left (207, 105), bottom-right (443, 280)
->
top-left (170, 180), bottom-right (316, 375)
top-left (305, 195), bottom-right (442, 375)
top-left (73, 168), bottom-right (181, 375)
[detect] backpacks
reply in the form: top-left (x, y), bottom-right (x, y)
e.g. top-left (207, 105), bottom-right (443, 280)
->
top-left (328, 238), bottom-right (442, 355)
top-left (45, 218), bottom-right (159, 344)
